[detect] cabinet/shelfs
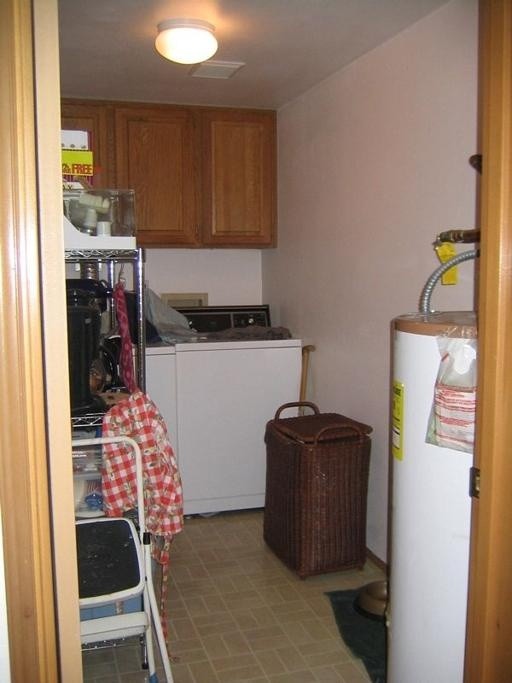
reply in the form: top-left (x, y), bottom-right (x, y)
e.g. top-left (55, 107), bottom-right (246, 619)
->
top-left (65, 248), bottom-right (146, 421)
top-left (62, 96), bottom-right (277, 248)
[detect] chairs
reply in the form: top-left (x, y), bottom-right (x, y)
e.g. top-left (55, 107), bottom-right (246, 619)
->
top-left (70, 439), bottom-right (175, 681)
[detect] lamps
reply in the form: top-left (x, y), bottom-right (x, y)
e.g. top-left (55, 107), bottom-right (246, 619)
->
top-left (154, 18), bottom-right (218, 64)
